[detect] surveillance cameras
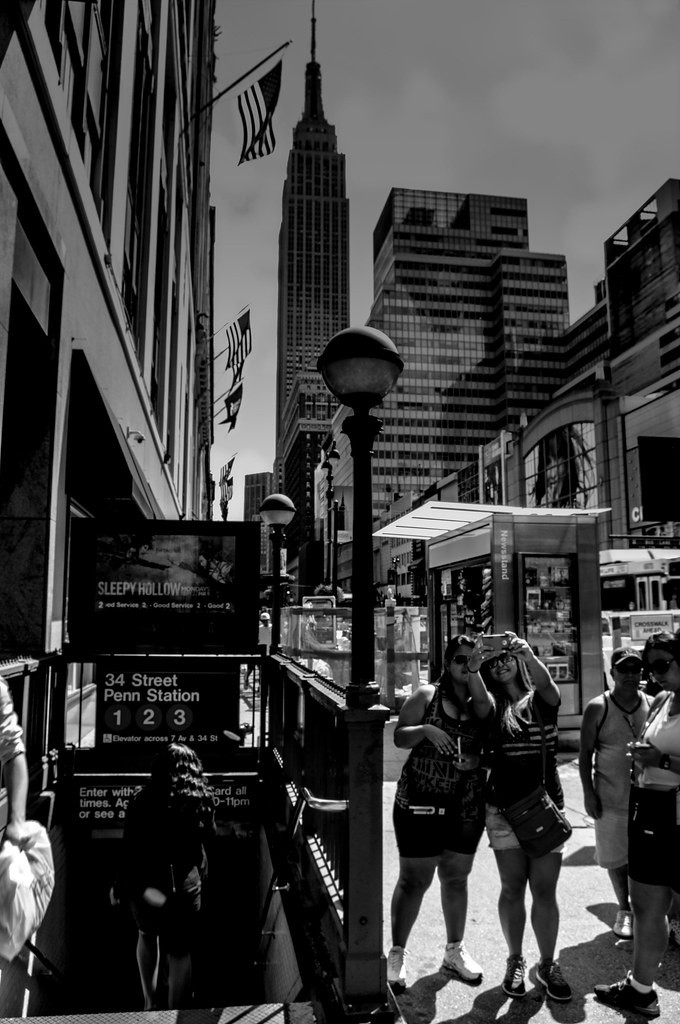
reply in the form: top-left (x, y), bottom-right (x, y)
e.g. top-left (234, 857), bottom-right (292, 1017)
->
top-left (133, 434), bottom-right (146, 443)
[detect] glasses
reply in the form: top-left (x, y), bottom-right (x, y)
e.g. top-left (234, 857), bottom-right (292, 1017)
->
top-left (450, 655), bottom-right (470, 664)
top-left (646, 657), bottom-right (676, 676)
top-left (487, 653), bottom-right (514, 669)
top-left (613, 664), bottom-right (642, 674)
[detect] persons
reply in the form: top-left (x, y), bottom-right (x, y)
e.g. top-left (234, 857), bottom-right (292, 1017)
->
top-left (387, 634), bottom-right (495, 995)
top-left (244, 612), bottom-right (273, 697)
top-left (593, 633), bottom-right (680, 1015)
top-left (109, 741), bottom-right (216, 1011)
top-left (468, 632), bottom-right (572, 1002)
top-left (579, 647), bottom-right (680, 946)
top-left (0, 675), bottom-right (31, 851)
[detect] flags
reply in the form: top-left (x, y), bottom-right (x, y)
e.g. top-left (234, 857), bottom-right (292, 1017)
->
top-left (238, 59), bottom-right (283, 166)
top-left (218, 310), bottom-right (253, 521)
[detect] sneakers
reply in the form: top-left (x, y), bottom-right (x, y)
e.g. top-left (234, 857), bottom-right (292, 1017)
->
top-left (669, 919), bottom-right (680, 944)
top-left (536, 960), bottom-right (573, 1000)
top-left (502, 956), bottom-right (528, 996)
top-left (594, 971), bottom-right (658, 1014)
top-left (443, 942), bottom-right (483, 980)
top-left (386, 946), bottom-right (409, 986)
top-left (613, 910), bottom-right (633, 937)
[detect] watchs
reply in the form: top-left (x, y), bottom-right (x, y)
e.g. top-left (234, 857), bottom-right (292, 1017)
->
top-left (660, 752), bottom-right (672, 769)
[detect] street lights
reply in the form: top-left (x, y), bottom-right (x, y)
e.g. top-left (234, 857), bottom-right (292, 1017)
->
top-left (320, 439), bottom-right (340, 595)
top-left (257, 492), bottom-right (297, 653)
top-left (320, 328), bottom-right (405, 682)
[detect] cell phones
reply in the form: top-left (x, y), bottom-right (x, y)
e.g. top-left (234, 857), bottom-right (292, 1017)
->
top-left (481, 634), bottom-right (512, 650)
top-left (635, 744), bottom-right (651, 750)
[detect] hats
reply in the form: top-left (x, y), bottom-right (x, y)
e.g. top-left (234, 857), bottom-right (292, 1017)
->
top-left (611, 647), bottom-right (642, 665)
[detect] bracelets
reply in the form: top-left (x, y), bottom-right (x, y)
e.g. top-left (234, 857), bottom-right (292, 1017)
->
top-left (468, 667), bottom-right (479, 674)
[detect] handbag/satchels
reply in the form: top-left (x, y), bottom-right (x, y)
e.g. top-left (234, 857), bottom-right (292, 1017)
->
top-left (502, 787), bottom-right (572, 860)
